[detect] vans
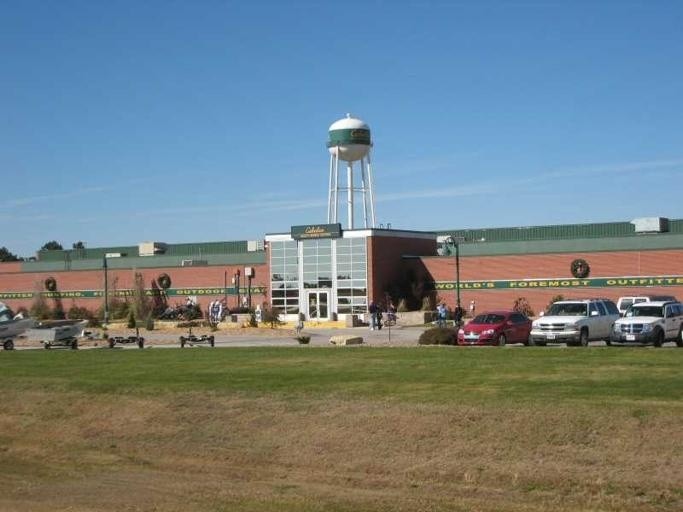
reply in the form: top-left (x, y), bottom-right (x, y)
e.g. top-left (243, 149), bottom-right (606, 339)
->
top-left (616, 296), bottom-right (676, 316)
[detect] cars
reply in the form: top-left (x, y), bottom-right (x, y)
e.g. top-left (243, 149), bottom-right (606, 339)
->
top-left (458, 311), bottom-right (533, 346)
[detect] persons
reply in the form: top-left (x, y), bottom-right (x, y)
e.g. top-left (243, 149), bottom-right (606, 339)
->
top-left (438, 303), bottom-right (448, 328)
top-left (384, 303), bottom-right (397, 325)
top-left (454, 303), bottom-right (462, 328)
top-left (375, 302), bottom-right (382, 330)
top-left (368, 299), bottom-right (376, 331)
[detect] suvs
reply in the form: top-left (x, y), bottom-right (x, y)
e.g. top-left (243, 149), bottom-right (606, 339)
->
top-left (529, 298), bottom-right (621, 346)
top-left (612, 300), bottom-right (683, 348)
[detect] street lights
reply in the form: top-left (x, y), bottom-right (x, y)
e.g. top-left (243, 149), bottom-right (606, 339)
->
top-left (442, 236), bottom-right (460, 307)
top-left (102, 256), bottom-right (110, 323)
